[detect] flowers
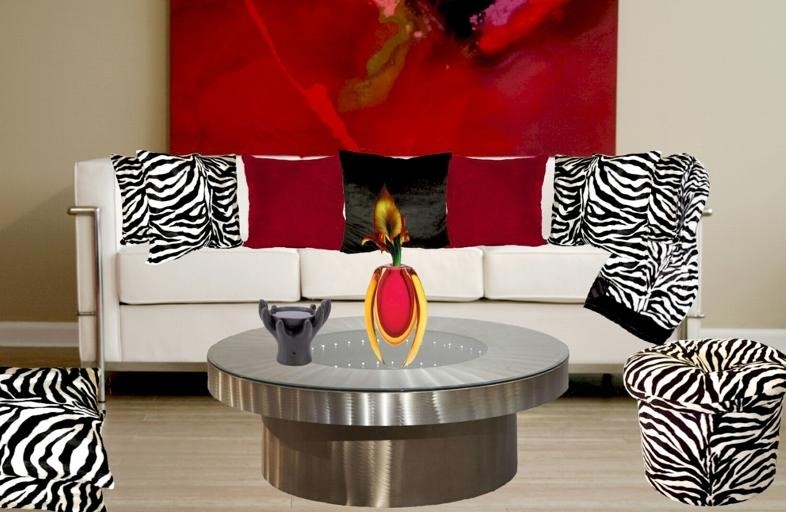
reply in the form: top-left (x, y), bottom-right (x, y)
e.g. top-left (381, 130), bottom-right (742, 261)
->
top-left (360, 185), bottom-right (412, 265)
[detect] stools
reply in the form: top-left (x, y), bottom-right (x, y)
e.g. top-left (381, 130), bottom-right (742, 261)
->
top-left (623, 336), bottom-right (786, 506)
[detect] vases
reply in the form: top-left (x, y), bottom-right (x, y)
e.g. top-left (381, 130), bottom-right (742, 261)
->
top-left (362, 264), bottom-right (428, 367)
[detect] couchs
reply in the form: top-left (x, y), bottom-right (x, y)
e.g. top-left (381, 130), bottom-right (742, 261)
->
top-left (67, 151), bottom-right (714, 400)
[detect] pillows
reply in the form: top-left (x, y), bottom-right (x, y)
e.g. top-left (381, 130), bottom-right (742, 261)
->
top-left (1, 396), bottom-right (116, 490)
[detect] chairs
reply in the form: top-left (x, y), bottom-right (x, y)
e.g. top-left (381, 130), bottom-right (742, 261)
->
top-left (0, 366), bottom-right (106, 511)
